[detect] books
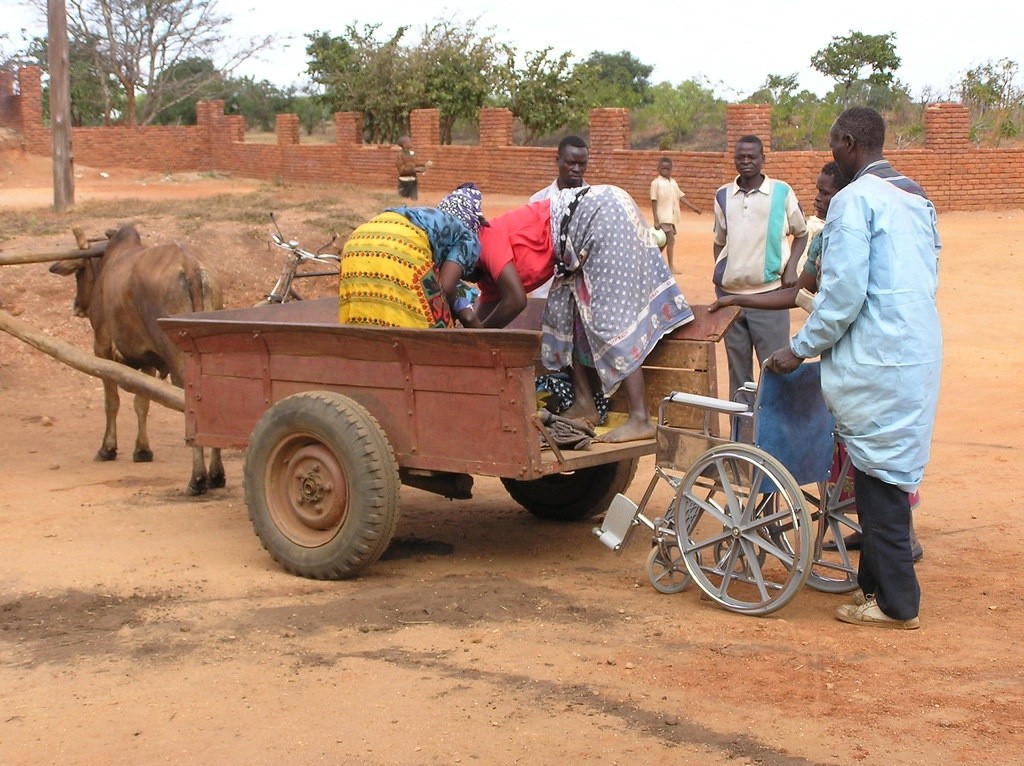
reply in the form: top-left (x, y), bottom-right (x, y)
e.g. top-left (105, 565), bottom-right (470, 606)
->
top-left (795, 286), bottom-right (817, 315)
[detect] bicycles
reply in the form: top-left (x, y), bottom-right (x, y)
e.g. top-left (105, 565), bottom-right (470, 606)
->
top-left (249, 211), bottom-right (343, 308)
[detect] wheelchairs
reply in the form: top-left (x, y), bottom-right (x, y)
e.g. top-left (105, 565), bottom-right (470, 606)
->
top-left (591, 356), bottom-right (861, 616)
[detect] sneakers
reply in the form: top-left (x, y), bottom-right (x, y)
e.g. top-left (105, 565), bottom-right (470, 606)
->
top-left (836, 590), bottom-right (921, 629)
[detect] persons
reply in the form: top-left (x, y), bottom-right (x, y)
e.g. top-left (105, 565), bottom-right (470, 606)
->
top-left (340, 135), bottom-right (696, 443)
top-left (766, 105), bottom-right (942, 629)
top-left (713, 135), bottom-right (809, 426)
top-left (707, 160), bottom-right (922, 565)
top-left (395, 136), bottom-right (427, 201)
top-left (649, 158), bottom-right (702, 274)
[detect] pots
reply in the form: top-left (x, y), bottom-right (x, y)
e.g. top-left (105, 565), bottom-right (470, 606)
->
top-left (651, 227), bottom-right (667, 248)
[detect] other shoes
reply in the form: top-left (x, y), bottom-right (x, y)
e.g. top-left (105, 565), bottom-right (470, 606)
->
top-left (911, 540), bottom-right (925, 561)
top-left (821, 532), bottom-right (863, 552)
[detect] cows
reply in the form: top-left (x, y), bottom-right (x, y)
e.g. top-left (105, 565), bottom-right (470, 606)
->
top-left (48, 225), bottom-right (226, 496)
